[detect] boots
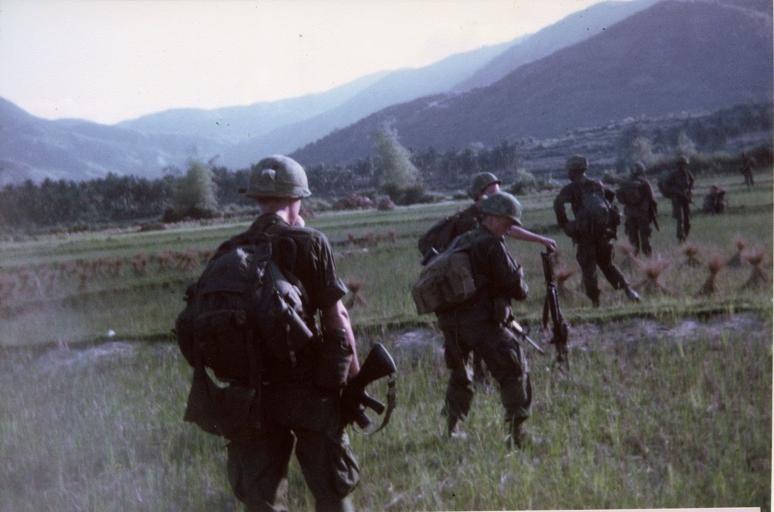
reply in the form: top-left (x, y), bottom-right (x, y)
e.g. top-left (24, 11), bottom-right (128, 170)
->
top-left (447, 416), bottom-right (468, 439)
top-left (592, 298), bottom-right (599, 307)
top-left (625, 286), bottom-right (641, 301)
top-left (509, 423), bottom-right (528, 449)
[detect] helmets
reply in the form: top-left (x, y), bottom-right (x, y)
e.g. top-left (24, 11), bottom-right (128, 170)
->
top-left (566, 156), bottom-right (587, 176)
top-left (632, 161), bottom-right (645, 176)
top-left (469, 172), bottom-right (522, 227)
top-left (245, 155), bottom-right (312, 199)
top-left (677, 155), bottom-right (689, 165)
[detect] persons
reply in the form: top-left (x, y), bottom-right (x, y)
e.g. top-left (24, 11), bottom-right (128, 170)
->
top-left (742, 152), bottom-right (755, 184)
top-left (702, 185), bottom-right (726, 212)
top-left (416, 173), bottom-right (559, 448)
top-left (184, 155), bottom-right (361, 512)
top-left (671, 156), bottom-right (695, 240)
top-left (554, 155), bottom-right (659, 308)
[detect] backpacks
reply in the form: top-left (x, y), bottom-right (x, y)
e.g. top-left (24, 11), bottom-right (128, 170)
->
top-left (614, 182), bottom-right (643, 205)
top-left (578, 190), bottom-right (609, 226)
top-left (412, 247), bottom-right (477, 316)
top-left (187, 237), bottom-right (313, 383)
top-left (657, 169), bottom-right (676, 199)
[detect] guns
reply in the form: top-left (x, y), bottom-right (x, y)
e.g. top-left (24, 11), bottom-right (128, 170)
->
top-left (338, 342), bottom-right (396, 430)
top-left (540, 248), bottom-right (570, 360)
